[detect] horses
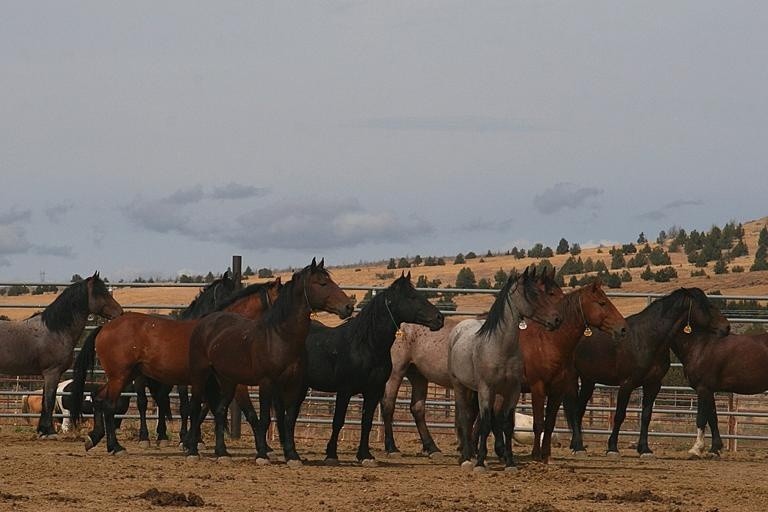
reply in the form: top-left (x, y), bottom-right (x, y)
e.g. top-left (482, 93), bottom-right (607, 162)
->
top-left (0, 256), bottom-right (767, 474)
